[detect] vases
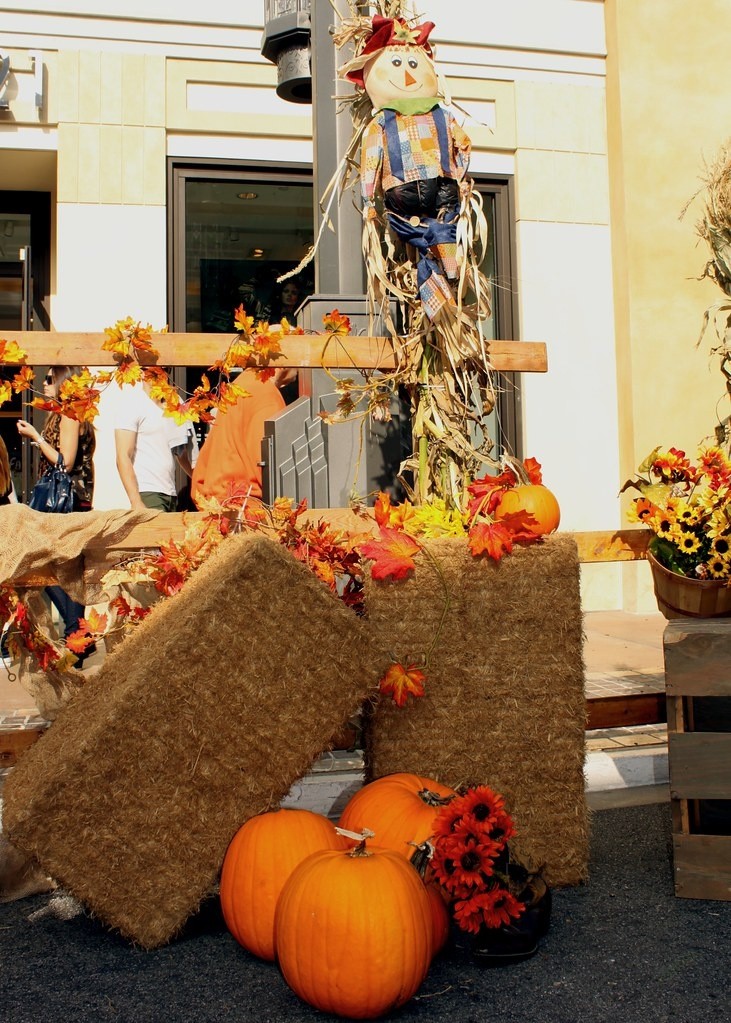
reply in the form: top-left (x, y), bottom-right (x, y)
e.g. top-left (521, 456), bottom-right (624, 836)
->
top-left (449, 876), bottom-right (552, 967)
top-left (646, 538), bottom-right (731, 623)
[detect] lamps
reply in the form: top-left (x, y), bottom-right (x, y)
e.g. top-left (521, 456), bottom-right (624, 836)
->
top-left (2, 221), bottom-right (13, 236)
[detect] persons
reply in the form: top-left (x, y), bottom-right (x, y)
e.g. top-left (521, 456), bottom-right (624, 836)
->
top-left (279, 281), bottom-right (298, 327)
top-left (0, 437), bottom-right (16, 659)
top-left (17, 365), bottom-right (97, 671)
top-left (115, 368), bottom-right (193, 606)
top-left (191, 324), bottom-right (298, 512)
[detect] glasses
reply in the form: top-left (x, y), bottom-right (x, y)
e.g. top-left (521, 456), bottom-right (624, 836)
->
top-left (45, 375), bottom-right (54, 385)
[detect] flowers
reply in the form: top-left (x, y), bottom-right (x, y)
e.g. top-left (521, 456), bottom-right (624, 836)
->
top-left (619, 446), bottom-right (731, 580)
top-left (428, 787), bottom-right (528, 934)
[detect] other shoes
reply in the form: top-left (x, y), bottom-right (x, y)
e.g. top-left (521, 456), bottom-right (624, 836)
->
top-left (83, 633), bottom-right (98, 658)
top-left (71, 654), bottom-right (84, 670)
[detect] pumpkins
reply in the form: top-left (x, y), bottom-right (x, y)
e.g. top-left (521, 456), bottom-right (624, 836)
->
top-left (493, 456), bottom-right (560, 536)
top-left (220, 772), bottom-right (464, 1019)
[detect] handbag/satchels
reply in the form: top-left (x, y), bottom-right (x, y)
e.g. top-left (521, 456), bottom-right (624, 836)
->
top-left (29, 453), bottom-right (74, 515)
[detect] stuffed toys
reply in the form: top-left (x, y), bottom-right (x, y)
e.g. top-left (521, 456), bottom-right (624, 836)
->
top-left (348, 14), bottom-right (471, 314)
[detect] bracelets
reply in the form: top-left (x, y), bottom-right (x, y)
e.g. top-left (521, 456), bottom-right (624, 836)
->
top-left (31, 437), bottom-right (45, 450)
